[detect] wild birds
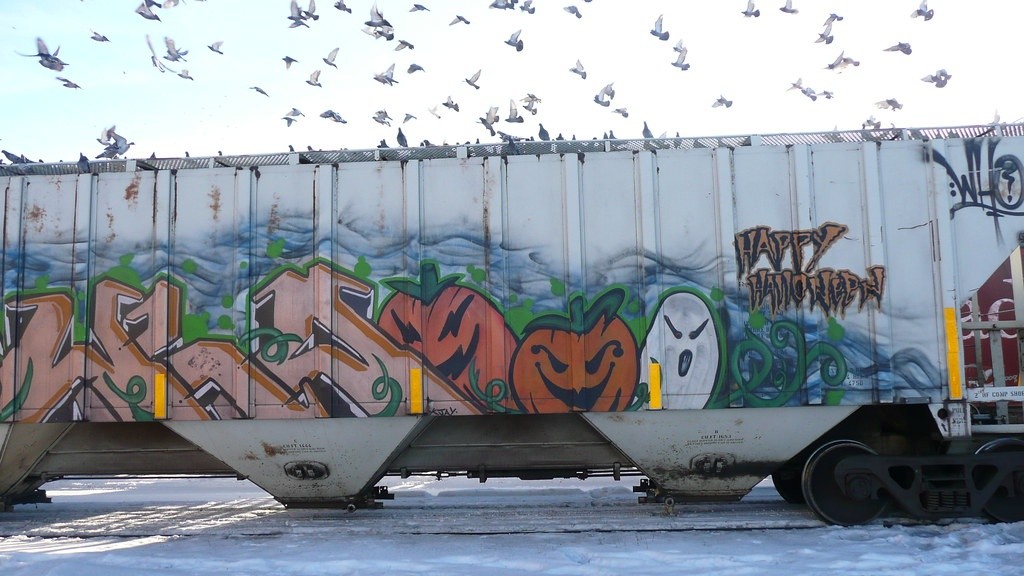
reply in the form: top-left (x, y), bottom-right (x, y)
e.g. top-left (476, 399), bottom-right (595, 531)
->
top-left (0, 0), bottom-right (1024, 173)
